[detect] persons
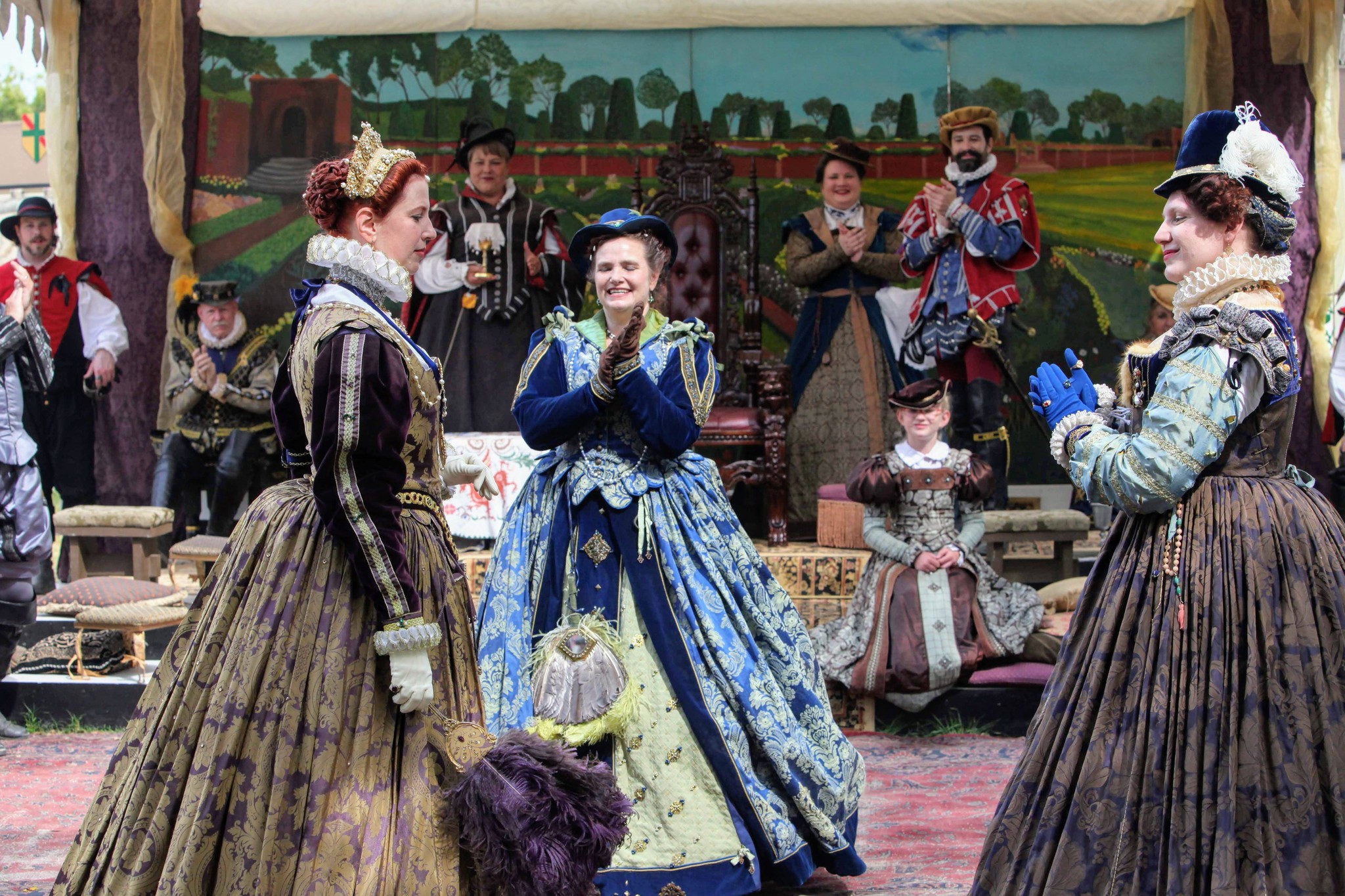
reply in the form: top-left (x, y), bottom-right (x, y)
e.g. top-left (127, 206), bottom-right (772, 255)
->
top-left (894, 106), bottom-right (1040, 515)
top-left (48, 117), bottom-right (485, 896)
top-left (401, 122), bottom-right (569, 433)
top-left (1139, 282), bottom-right (1178, 338)
top-left (1, 194), bottom-right (130, 508)
top-left (969, 105), bottom-right (1344, 896)
top-left (810, 374), bottom-right (1065, 727)
top-left (149, 280), bottom-right (291, 566)
top-left (0, 258), bottom-right (57, 738)
top-left (779, 138), bottom-right (904, 534)
top-left (475, 207), bottom-right (870, 894)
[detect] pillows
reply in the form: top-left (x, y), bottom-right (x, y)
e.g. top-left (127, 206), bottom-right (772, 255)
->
top-left (11, 628), bottom-right (126, 675)
top-left (35, 574), bottom-right (187, 616)
top-left (1038, 576), bottom-right (1087, 612)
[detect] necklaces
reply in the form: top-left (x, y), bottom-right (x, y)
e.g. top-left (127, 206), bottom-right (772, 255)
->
top-left (606, 327), bottom-right (616, 342)
top-left (328, 275), bottom-right (411, 339)
top-left (308, 301), bottom-right (446, 420)
top-left (1211, 283), bottom-right (1267, 304)
top-left (567, 326), bottom-right (667, 483)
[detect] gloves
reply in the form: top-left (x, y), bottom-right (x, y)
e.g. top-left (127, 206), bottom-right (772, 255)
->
top-left (615, 300), bottom-right (647, 363)
top-left (1037, 362), bottom-right (1093, 432)
top-left (1029, 348), bottom-right (1100, 417)
top-left (598, 326), bottom-right (628, 388)
top-left (388, 648), bottom-right (434, 713)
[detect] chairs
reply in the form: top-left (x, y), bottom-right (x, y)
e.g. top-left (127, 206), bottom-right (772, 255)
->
top-left (152, 441), bottom-right (270, 546)
top-left (628, 119), bottom-right (793, 550)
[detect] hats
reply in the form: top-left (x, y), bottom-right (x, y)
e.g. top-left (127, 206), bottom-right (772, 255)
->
top-left (568, 208), bottom-right (678, 285)
top-left (888, 378), bottom-right (952, 410)
top-left (821, 136), bottom-right (872, 167)
top-left (938, 106), bottom-right (1001, 149)
top-left (446, 117), bottom-right (516, 172)
top-left (0, 197), bottom-right (57, 247)
top-left (1153, 101), bottom-right (1306, 255)
top-left (193, 281), bottom-right (240, 306)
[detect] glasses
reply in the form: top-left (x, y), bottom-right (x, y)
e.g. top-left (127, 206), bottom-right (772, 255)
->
top-left (902, 407), bottom-right (943, 417)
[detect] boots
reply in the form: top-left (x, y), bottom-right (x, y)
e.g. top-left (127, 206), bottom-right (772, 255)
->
top-left (206, 467), bottom-right (242, 537)
top-left (150, 455), bottom-right (176, 568)
top-left (0, 589), bottom-right (37, 739)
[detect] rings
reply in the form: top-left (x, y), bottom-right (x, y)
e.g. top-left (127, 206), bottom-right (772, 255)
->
top-left (391, 683), bottom-right (401, 693)
top-left (1041, 399), bottom-right (1051, 408)
top-left (1069, 360), bottom-right (1084, 370)
top-left (1063, 380), bottom-right (1071, 389)
top-left (95, 375), bottom-right (102, 380)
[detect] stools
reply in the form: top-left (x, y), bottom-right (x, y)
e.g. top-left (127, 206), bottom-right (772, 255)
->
top-left (980, 508), bottom-right (1090, 578)
top-left (816, 483), bottom-right (879, 550)
top-left (1057, 550), bottom-right (1066, 554)
top-left (52, 504), bottom-right (231, 671)
top-left (874, 660), bottom-right (1057, 719)
